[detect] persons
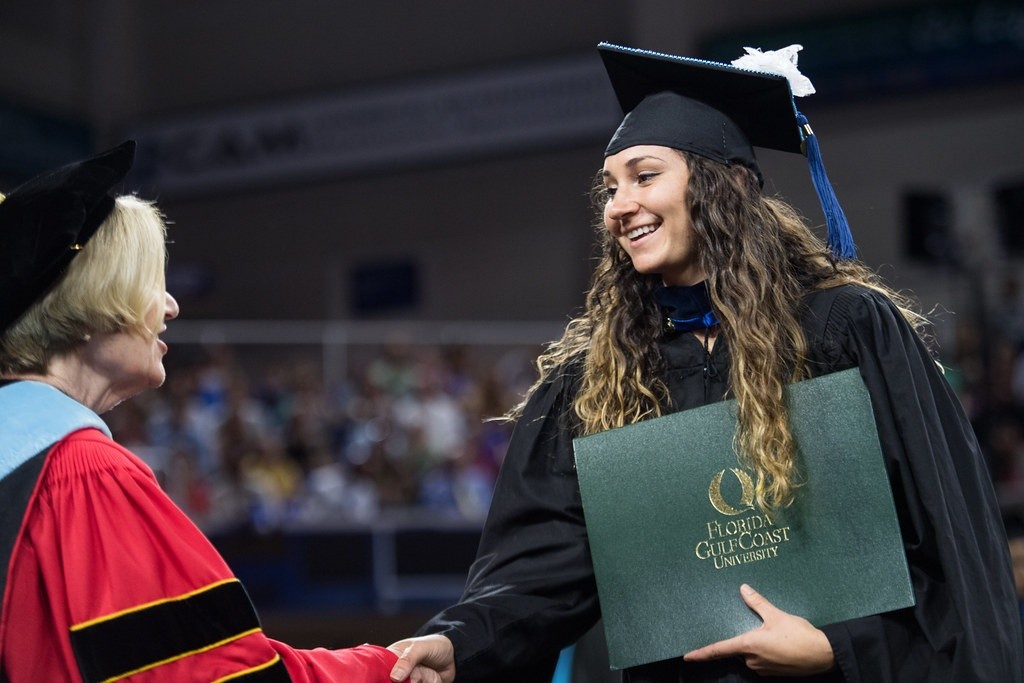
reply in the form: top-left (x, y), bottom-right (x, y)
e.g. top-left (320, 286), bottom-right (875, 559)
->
top-left (1, 139), bottom-right (446, 683)
top-left (388, 38), bottom-right (1024, 683)
top-left (107, 311), bottom-right (1024, 528)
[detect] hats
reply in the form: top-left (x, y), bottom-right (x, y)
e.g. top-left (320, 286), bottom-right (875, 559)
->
top-left (595, 39), bottom-right (856, 261)
top-left (0, 138), bottom-right (137, 337)
